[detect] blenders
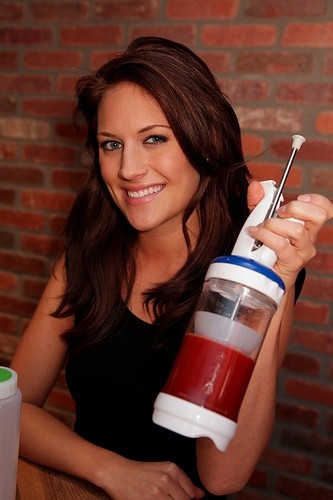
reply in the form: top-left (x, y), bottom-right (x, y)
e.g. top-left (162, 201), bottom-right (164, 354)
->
top-left (152, 133), bottom-right (305, 451)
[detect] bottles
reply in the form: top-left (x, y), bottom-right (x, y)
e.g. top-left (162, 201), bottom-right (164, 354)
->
top-left (0, 364), bottom-right (24, 500)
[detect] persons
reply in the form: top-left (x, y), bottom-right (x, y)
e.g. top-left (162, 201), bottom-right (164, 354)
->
top-left (9, 38), bottom-right (332, 500)
